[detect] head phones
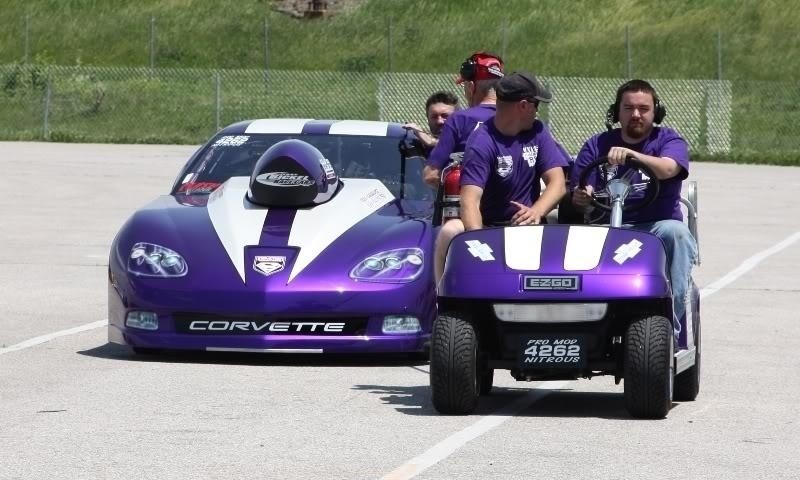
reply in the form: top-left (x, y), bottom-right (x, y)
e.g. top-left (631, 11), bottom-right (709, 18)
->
top-left (606, 86), bottom-right (667, 123)
top-left (460, 51), bottom-right (504, 81)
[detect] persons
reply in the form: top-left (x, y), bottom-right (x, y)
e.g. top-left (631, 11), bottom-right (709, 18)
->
top-left (402, 91), bottom-right (458, 163)
top-left (423, 52), bottom-right (505, 225)
top-left (432, 71), bottom-right (569, 294)
top-left (570, 81), bottom-right (696, 354)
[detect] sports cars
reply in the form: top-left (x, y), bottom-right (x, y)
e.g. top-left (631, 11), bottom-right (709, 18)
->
top-left (108, 117), bottom-right (444, 362)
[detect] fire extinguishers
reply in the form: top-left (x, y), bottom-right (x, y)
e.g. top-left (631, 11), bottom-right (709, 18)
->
top-left (440, 151), bottom-right (465, 224)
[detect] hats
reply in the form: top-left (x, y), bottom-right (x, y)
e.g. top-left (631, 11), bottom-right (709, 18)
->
top-left (455, 54), bottom-right (502, 86)
top-left (496, 71), bottom-right (553, 103)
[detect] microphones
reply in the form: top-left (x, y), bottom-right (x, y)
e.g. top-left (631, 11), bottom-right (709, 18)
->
top-left (605, 120), bottom-right (611, 131)
